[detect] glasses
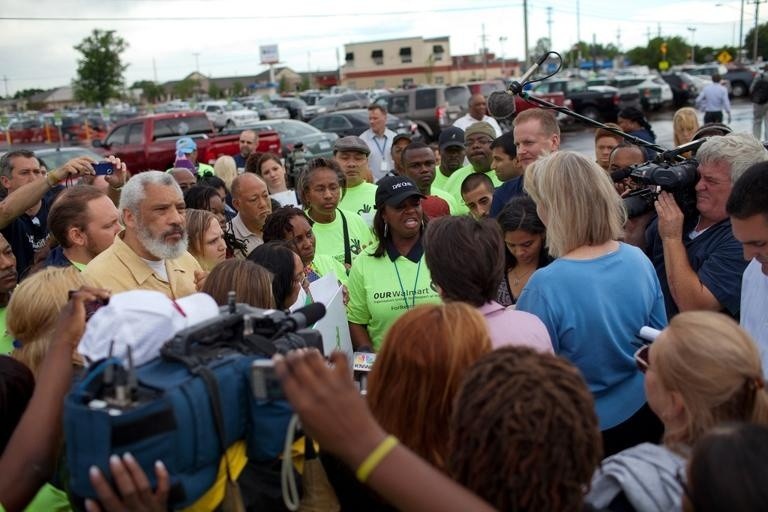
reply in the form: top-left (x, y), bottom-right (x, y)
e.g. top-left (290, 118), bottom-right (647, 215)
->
top-left (634, 344), bottom-right (660, 382)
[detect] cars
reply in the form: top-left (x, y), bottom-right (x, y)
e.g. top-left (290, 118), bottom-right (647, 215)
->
top-left (468, 60), bottom-right (767, 132)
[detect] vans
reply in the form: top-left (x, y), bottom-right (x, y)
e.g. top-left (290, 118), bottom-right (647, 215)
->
top-left (368, 83), bottom-right (475, 145)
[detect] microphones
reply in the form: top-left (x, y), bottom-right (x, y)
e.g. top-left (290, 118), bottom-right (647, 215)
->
top-left (485, 48), bottom-right (553, 122)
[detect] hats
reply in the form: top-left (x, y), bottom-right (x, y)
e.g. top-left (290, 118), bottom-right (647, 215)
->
top-left (375, 175), bottom-right (450, 220)
top-left (393, 133), bottom-right (412, 144)
top-left (333, 135), bottom-right (371, 157)
top-left (465, 122), bottom-right (497, 140)
top-left (439, 126), bottom-right (466, 151)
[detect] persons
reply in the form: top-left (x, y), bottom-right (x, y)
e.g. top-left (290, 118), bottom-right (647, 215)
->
top-left (1, 62), bottom-right (767, 512)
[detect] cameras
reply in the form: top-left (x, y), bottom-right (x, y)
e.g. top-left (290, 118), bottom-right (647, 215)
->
top-left (90, 162), bottom-right (114, 176)
